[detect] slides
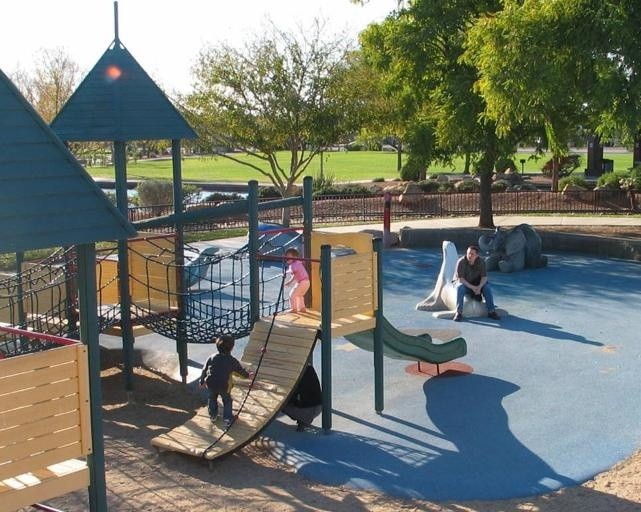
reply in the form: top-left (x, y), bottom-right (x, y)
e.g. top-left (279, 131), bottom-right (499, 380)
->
top-left (344, 309), bottom-right (467, 364)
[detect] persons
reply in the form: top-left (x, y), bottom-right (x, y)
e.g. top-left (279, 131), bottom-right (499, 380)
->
top-left (453, 244), bottom-right (501, 320)
top-left (198, 335), bottom-right (254, 428)
top-left (284, 248), bottom-right (311, 314)
top-left (282, 361), bottom-right (323, 432)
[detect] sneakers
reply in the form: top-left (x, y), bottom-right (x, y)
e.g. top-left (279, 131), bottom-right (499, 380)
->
top-left (453, 313), bottom-right (462, 321)
top-left (488, 311), bottom-right (502, 320)
